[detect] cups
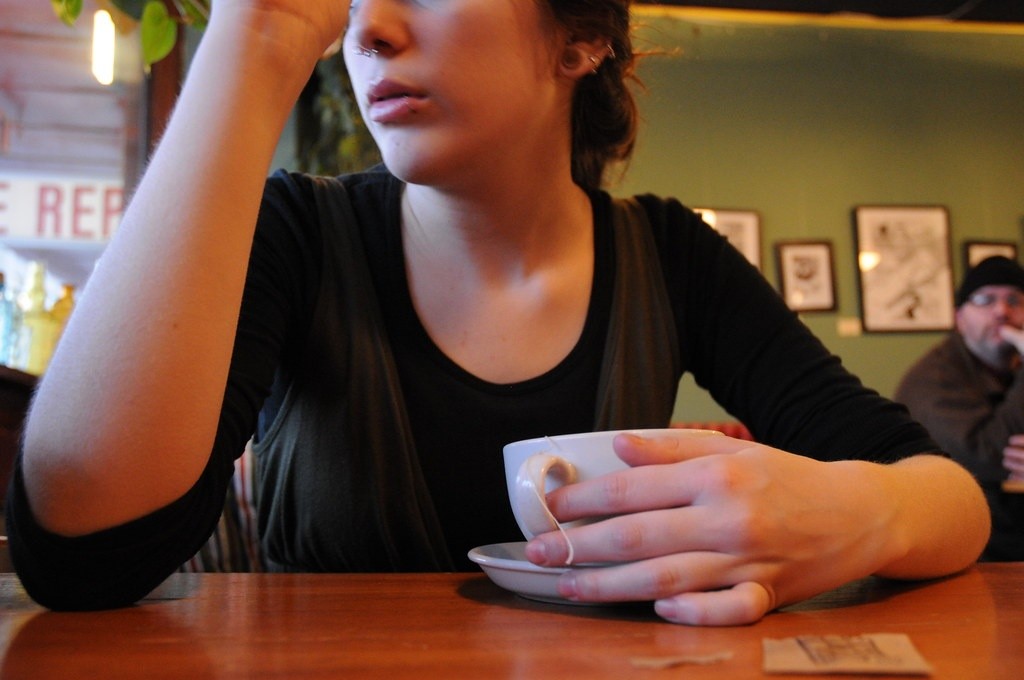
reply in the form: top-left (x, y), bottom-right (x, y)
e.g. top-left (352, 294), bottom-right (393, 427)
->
top-left (501, 428), bottom-right (726, 569)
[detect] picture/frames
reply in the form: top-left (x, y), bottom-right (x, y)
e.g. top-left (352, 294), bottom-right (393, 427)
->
top-left (691, 205), bottom-right (763, 272)
top-left (773, 239), bottom-right (836, 312)
top-left (852, 205), bottom-right (958, 333)
top-left (963, 240), bottom-right (1018, 270)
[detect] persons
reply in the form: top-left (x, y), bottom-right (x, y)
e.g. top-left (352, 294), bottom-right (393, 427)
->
top-left (6, 0), bottom-right (991, 627)
top-left (894, 253), bottom-right (1024, 561)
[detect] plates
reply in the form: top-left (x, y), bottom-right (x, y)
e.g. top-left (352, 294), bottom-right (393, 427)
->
top-left (468, 541), bottom-right (650, 607)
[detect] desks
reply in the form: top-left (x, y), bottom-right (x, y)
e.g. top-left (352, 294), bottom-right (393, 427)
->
top-left (0, 564), bottom-right (1024, 680)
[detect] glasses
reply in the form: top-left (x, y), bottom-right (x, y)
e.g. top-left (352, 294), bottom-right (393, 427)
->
top-left (966, 293), bottom-right (1024, 307)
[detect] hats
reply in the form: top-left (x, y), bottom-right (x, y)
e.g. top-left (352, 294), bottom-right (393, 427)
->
top-left (956, 256), bottom-right (1024, 307)
